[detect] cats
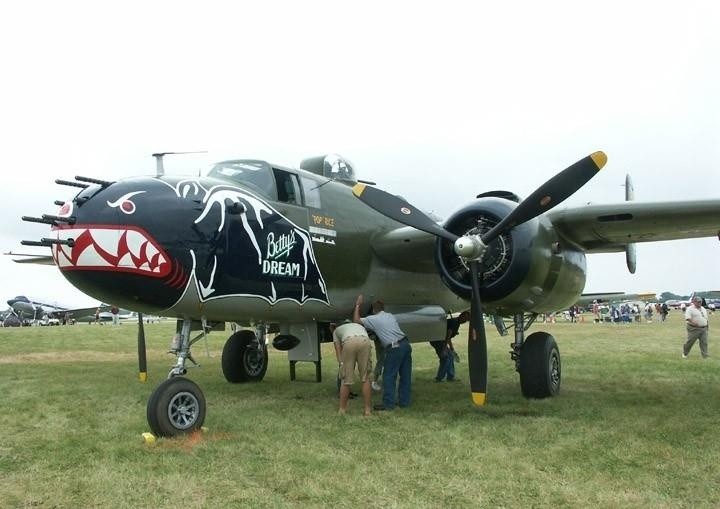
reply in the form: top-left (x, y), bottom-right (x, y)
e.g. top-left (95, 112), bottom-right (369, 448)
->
top-left (666, 299), bottom-right (720, 310)
top-left (0, 296), bottom-right (167, 328)
top-left (2, 149), bottom-right (720, 438)
top-left (574, 292), bottom-right (654, 308)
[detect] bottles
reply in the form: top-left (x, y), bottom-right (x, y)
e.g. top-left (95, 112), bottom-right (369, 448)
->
top-left (372, 301), bottom-right (384, 311)
top-left (334, 320), bottom-right (346, 327)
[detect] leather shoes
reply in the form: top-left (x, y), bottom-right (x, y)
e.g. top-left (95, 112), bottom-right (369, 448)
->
top-left (452, 350), bottom-right (460, 363)
top-left (439, 347), bottom-right (450, 359)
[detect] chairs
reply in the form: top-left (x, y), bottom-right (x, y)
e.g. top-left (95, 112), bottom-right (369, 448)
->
top-left (435, 376), bottom-right (461, 382)
top-left (363, 399), bottom-right (400, 417)
top-left (682, 351), bottom-right (687, 359)
top-left (371, 381), bottom-right (381, 391)
top-left (338, 391), bottom-right (358, 399)
top-left (337, 409), bottom-right (352, 416)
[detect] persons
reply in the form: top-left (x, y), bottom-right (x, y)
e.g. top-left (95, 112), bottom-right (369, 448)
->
top-left (94, 307), bottom-right (101, 325)
top-left (333, 319), bottom-right (375, 417)
top-left (428, 311), bottom-right (472, 383)
top-left (542, 298), bottom-right (671, 324)
top-left (372, 348), bottom-right (386, 391)
top-left (681, 295), bottom-right (714, 359)
top-left (352, 294), bottom-right (413, 411)
top-left (111, 305), bottom-right (120, 325)
top-left (328, 322), bottom-right (359, 400)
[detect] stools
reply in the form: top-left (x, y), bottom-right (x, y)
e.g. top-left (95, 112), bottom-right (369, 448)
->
top-left (687, 323), bottom-right (707, 328)
top-left (389, 336), bottom-right (407, 349)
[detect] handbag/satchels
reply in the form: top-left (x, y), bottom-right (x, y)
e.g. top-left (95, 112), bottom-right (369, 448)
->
top-left (338, 360), bottom-right (344, 367)
top-left (355, 304), bottom-right (360, 308)
